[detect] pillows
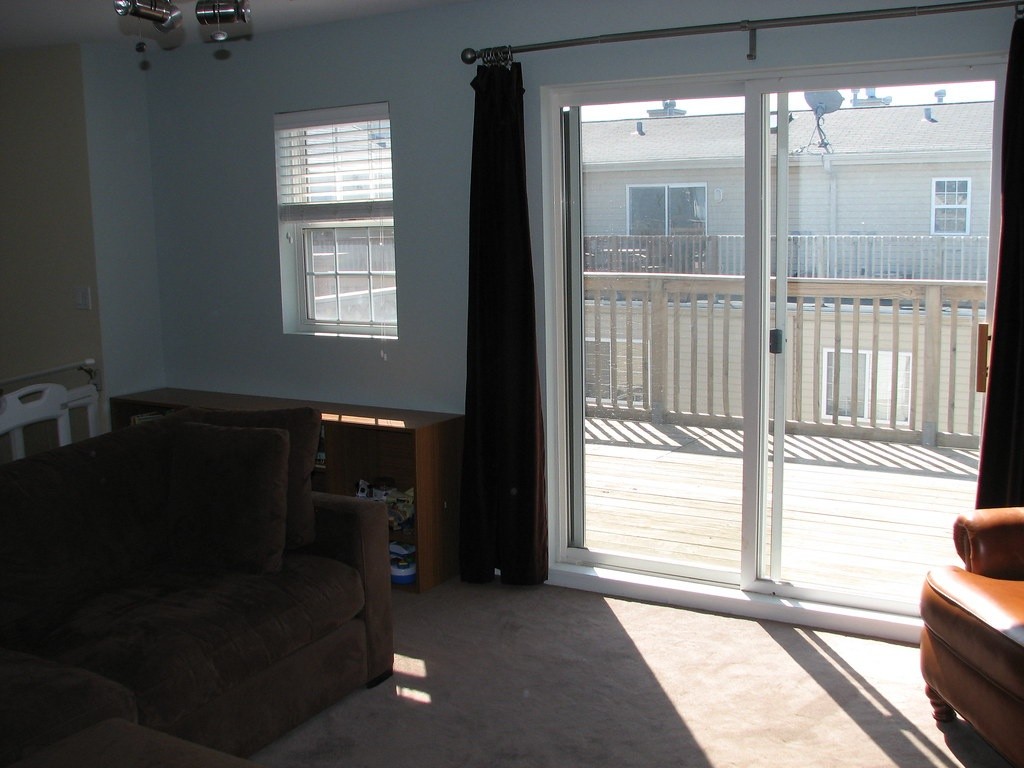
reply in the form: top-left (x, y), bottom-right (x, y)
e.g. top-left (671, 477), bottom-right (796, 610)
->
top-left (195, 407), bottom-right (323, 552)
top-left (163, 426), bottom-right (289, 575)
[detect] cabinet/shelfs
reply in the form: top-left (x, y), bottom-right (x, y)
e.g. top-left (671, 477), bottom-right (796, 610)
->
top-left (109, 388), bottom-right (465, 594)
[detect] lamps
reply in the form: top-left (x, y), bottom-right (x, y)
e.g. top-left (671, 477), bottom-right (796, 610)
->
top-left (114, 0), bottom-right (183, 51)
top-left (195, 0), bottom-right (253, 44)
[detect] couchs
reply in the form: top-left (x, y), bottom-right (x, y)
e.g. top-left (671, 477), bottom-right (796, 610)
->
top-left (922, 506), bottom-right (1024, 768)
top-left (0, 422), bottom-right (394, 768)
